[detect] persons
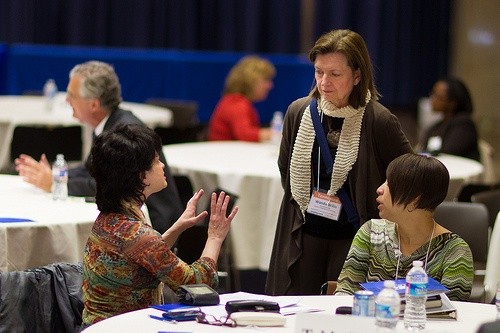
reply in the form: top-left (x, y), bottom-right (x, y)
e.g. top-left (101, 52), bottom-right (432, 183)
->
top-left (418, 77), bottom-right (485, 203)
top-left (266, 29), bottom-right (415, 295)
top-left (332, 154), bottom-right (473, 301)
top-left (207, 57), bottom-right (282, 142)
top-left (81, 123), bottom-right (238, 331)
top-left (13, 61), bottom-right (184, 245)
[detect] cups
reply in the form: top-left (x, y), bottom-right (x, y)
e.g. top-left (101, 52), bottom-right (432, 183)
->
top-left (426, 137), bottom-right (442, 157)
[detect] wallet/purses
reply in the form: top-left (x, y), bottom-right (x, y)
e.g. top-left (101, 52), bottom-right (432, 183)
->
top-left (176, 283), bottom-right (220, 306)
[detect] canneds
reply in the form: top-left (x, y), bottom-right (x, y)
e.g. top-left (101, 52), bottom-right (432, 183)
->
top-left (352, 290), bottom-right (375, 317)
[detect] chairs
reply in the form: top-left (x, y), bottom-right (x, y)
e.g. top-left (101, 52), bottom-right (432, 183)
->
top-left (172, 175), bottom-right (197, 218)
top-left (145, 97), bottom-right (197, 127)
top-left (478, 139), bottom-right (494, 183)
top-left (456, 180), bottom-right (500, 203)
top-left (9, 124), bottom-right (83, 177)
top-left (171, 187), bottom-right (240, 293)
top-left (430, 199), bottom-right (496, 305)
top-left (0, 261), bottom-right (85, 333)
top-left (469, 189), bottom-right (500, 235)
top-left (153, 121), bottom-right (207, 146)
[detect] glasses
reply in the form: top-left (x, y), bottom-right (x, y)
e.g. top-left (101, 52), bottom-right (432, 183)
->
top-left (195, 306), bottom-right (236, 328)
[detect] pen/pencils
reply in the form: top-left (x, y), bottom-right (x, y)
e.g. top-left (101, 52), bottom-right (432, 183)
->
top-left (148, 315), bottom-right (176, 323)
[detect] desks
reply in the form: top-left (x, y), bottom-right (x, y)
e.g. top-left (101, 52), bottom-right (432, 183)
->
top-left (0, 93), bottom-right (500, 333)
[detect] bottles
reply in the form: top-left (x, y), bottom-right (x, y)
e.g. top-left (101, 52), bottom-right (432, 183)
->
top-left (52, 154), bottom-right (69, 203)
top-left (44, 78), bottom-right (56, 113)
top-left (270, 111), bottom-right (284, 146)
top-left (402, 260), bottom-right (428, 332)
top-left (373, 280), bottom-right (400, 333)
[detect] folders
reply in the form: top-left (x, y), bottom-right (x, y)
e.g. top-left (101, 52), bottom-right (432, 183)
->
top-left (400, 292), bottom-right (457, 321)
top-left (359, 274), bottom-right (451, 298)
